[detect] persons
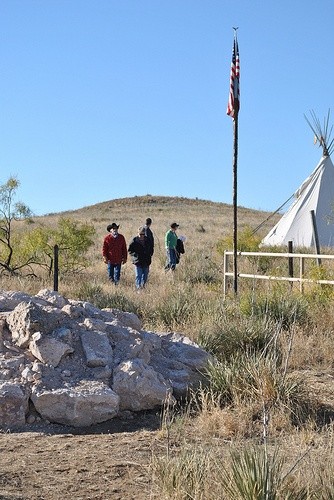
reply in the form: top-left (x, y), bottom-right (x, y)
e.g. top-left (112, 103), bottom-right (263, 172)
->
top-left (103, 223), bottom-right (128, 286)
top-left (164, 223), bottom-right (180, 275)
top-left (128, 227), bottom-right (154, 289)
top-left (141, 218), bottom-right (153, 256)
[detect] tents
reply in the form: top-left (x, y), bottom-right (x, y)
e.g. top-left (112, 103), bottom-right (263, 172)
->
top-left (258, 143), bottom-right (334, 248)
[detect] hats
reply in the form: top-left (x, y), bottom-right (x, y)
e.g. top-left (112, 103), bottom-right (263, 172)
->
top-left (170, 223), bottom-right (179, 227)
top-left (106, 223), bottom-right (120, 232)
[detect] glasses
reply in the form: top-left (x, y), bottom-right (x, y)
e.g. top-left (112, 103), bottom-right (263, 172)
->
top-left (139, 233), bottom-right (145, 235)
